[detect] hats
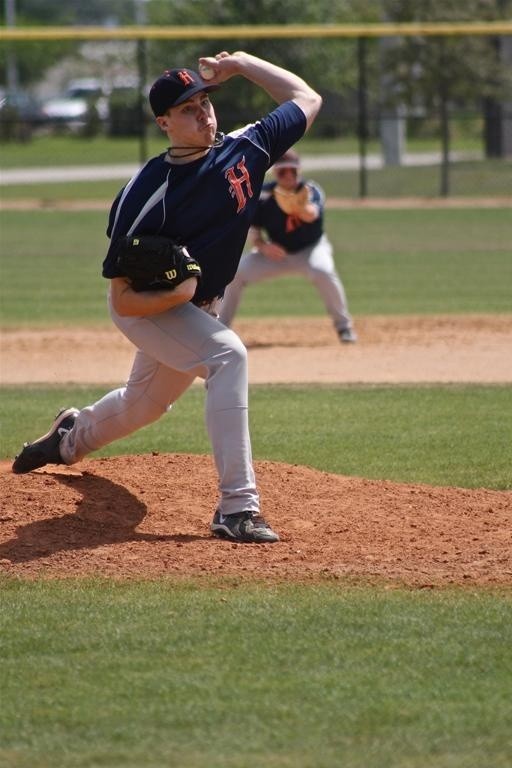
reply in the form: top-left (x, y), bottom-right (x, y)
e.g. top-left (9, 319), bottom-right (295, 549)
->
top-left (274, 148), bottom-right (300, 170)
top-left (149, 68), bottom-right (220, 118)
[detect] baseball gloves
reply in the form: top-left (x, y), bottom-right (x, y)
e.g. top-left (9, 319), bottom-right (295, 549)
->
top-left (125, 236), bottom-right (202, 293)
top-left (273, 185), bottom-right (313, 215)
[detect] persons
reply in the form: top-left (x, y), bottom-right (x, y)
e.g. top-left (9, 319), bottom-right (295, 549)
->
top-left (211, 145), bottom-right (359, 345)
top-left (13, 49), bottom-right (324, 544)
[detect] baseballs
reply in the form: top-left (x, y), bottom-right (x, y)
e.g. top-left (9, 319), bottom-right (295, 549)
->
top-left (199, 57), bottom-right (218, 80)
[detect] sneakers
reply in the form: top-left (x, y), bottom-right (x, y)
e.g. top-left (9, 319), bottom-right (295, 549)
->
top-left (213, 509), bottom-right (279, 542)
top-left (338, 329), bottom-right (357, 343)
top-left (12, 407), bottom-right (81, 474)
